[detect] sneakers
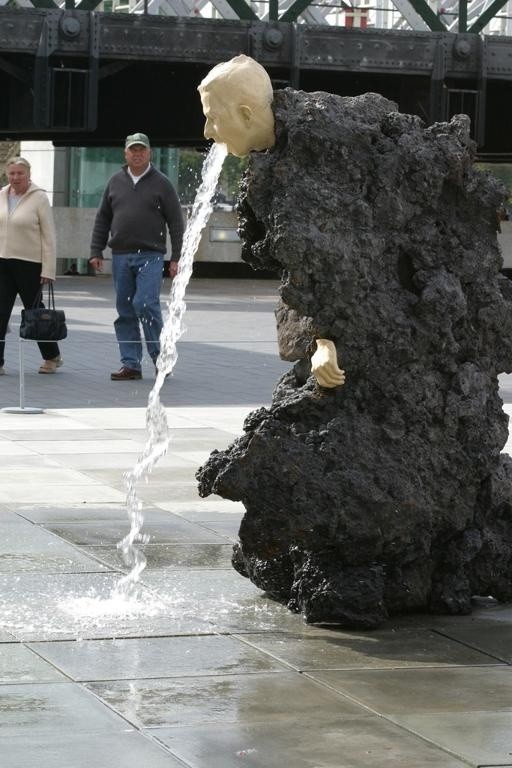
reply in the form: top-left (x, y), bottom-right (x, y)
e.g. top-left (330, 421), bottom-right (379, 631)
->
top-left (0, 353), bottom-right (64, 374)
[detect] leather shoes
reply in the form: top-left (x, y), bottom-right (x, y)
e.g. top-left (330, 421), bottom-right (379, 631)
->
top-left (111, 362), bottom-right (172, 381)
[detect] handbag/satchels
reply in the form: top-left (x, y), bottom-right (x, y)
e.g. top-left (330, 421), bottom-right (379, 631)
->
top-left (19, 279), bottom-right (67, 341)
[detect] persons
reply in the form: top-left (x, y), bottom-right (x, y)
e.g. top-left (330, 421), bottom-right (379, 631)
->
top-left (0, 157), bottom-right (64, 375)
top-left (89, 133), bottom-right (184, 380)
top-left (196, 54), bottom-right (345, 388)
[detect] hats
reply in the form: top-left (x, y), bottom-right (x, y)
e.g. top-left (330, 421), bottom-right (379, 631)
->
top-left (125, 133), bottom-right (150, 150)
